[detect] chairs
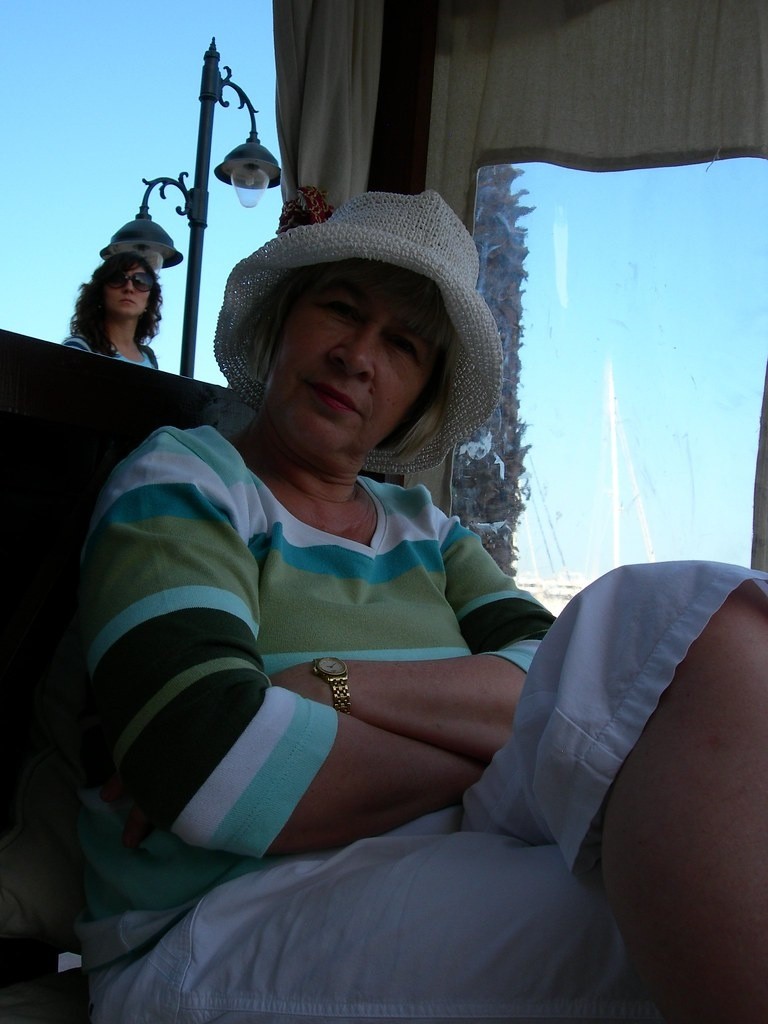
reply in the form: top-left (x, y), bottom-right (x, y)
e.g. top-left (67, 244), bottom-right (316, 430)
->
top-left (0, 331), bottom-right (404, 1024)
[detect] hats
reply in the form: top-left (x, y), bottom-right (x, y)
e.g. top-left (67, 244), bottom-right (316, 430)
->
top-left (214, 189), bottom-right (504, 476)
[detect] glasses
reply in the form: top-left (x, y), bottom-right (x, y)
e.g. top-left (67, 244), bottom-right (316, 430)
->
top-left (106, 271), bottom-right (154, 292)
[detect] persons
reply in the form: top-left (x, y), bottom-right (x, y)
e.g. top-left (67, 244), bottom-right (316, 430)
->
top-left (62, 249), bottom-right (162, 370)
top-left (65, 190), bottom-right (768, 1024)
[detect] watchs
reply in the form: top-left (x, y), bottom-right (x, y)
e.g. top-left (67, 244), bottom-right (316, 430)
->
top-left (312, 655), bottom-right (352, 716)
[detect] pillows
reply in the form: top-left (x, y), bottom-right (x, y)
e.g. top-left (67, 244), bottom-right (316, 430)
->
top-left (0, 606), bottom-right (86, 953)
